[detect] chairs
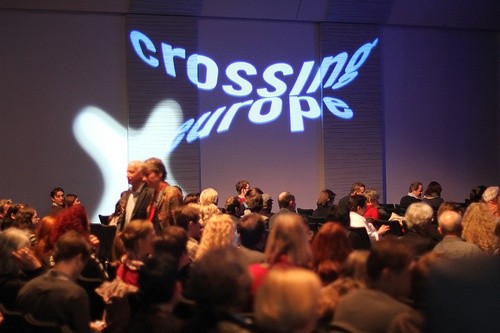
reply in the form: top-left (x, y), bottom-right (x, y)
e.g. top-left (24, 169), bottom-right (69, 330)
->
top-left (89, 204), bottom-right (407, 247)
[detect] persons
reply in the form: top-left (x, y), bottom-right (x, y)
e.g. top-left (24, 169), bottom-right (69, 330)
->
top-left (0, 180), bottom-right (497, 333)
top-left (142, 158), bottom-right (183, 234)
top-left (115, 160), bottom-right (152, 254)
